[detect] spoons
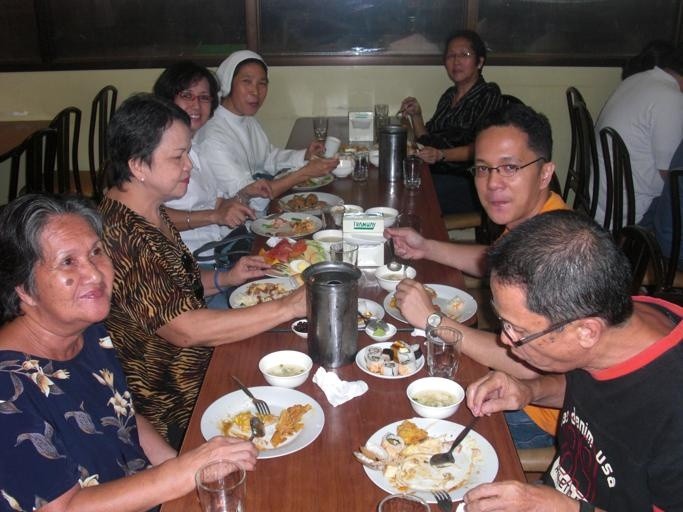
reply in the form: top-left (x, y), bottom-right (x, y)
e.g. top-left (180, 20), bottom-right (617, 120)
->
top-left (429, 415), bottom-right (481, 468)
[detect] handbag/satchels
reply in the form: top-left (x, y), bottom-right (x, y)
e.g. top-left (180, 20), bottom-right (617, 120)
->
top-left (190, 220), bottom-right (256, 269)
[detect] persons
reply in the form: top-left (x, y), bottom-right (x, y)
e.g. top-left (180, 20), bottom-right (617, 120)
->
top-left (383, 106), bottom-right (576, 449)
top-left (655, 138), bottom-right (683, 272)
top-left (152, 62), bottom-right (274, 309)
top-left (588, 45), bottom-right (683, 231)
top-left (194, 50), bottom-right (339, 211)
top-left (465, 209), bottom-right (683, 512)
top-left (90, 93), bottom-right (308, 453)
top-left (401, 29), bottom-right (502, 217)
top-left (0, 193), bottom-right (259, 512)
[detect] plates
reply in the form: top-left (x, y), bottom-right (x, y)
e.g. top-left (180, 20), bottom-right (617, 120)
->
top-left (361, 417), bottom-right (499, 502)
top-left (199, 191), bottom-right (496, 460)
top-left (410, 141), bottom-right (427, 158)
top-left (274, 171), bottom-right (334, 190)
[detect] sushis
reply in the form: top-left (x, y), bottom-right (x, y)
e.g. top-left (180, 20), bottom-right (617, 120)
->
top-left (364, 341), bottom-right (422, 376)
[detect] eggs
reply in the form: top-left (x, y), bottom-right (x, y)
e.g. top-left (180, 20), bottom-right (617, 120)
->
top-left (290, 259), bottom-right (311, 273)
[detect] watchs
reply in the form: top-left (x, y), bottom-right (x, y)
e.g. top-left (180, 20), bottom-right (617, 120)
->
top-left (425, 311), bottom-right (446, 337)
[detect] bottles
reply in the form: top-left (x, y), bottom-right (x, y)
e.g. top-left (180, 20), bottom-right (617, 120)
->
top-left (380, 125), bottom-right (409, 181)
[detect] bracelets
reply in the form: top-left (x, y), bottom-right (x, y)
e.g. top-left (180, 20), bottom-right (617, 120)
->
top-left (214, 268), bottom-right (226, 294)
top-left (185, 210), bottom-right (194, 230)
top-left (439, 150), bottom-right (445, 163)
top-left (233, 195), bottom-right (250, 204)
top-left (579, 498), bottom-right (595, 512)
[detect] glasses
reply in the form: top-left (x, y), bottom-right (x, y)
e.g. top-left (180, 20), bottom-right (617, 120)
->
top-left (178, 91), bottom-right (215, 103)
top-left (466, 157), bottom-right (549, 178)
top-left (486, 300), bottom-right (607, 350)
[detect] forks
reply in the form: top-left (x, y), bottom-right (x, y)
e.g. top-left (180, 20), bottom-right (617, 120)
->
top-left (431, 489), bottom-right (453, 511)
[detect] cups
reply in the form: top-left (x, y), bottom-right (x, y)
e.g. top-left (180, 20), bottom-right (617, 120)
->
top-left (312, 117), bottom-right (370, 181)
top-left (374, 103), bottom-right (389, 147)
top-left (194, 461), bottom-right (248, 512)
top-left (403, 158), bottom-right (424, 190)
top-left (375, 491), bottom-right (432, 511)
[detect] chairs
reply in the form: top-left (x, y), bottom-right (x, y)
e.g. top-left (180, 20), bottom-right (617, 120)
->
top-left (0, 85), bottom-right (119, 328)
top-left (443, 85), bottom-right (683, 475)
